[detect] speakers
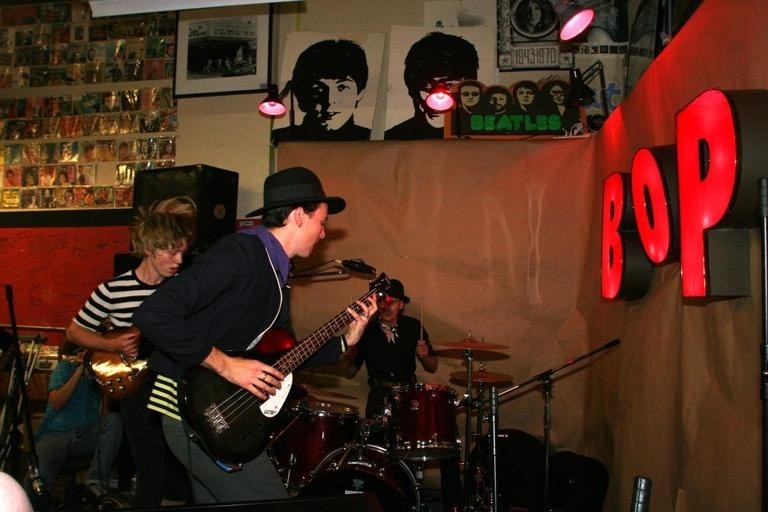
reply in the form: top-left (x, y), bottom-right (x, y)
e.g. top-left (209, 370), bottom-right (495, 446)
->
top-left (132, 164), bottom-right (239, 255)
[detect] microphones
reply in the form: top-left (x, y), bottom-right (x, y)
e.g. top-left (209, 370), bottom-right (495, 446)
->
top-left (448, 395), bottom-right (485, 408)
top-left (32, 479), bottom-right (44, 496)
top-left (334, 257), bottom-right (377, 274)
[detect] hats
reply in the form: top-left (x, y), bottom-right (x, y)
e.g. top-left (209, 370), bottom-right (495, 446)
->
top-left (387, 279), bottom-right (410, 303)
top-left (246, 167), bottom-right (346, 218)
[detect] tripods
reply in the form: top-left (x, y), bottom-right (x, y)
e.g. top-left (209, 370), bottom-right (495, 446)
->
top-left (438, 359), bottom-right (479, 511)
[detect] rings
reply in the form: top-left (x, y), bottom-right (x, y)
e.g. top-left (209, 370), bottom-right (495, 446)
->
top-left (364, 314), bottom-right (369, 317)
top-left (262, 372), bottom-right (268, 379)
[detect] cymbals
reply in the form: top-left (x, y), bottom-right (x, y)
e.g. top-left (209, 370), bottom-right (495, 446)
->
top-left (433, 341), bottom-right (510, 351)
top-left (450, 371), bottom-right (512, 382)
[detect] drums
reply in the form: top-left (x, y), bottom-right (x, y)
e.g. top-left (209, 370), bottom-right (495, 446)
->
top-left (305, 444), bottom-right (419, 512)
top-left (281, 400), bottom-right (361, 485)
top-left (386, 386), bottom-right (461, 460)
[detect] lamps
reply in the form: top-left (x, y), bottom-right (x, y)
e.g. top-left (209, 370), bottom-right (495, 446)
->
top-left (425, 79), bottom-right (462, 139)
top-left (568, 60), bottom-right (609, 126)
top-left (558, 4), bottom-right (594, 69)
top-left (257, 80), bottom-right (295, 141)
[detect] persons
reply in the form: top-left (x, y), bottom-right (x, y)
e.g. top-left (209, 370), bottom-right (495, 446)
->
top-left (149, 196), bottom-right (200, 249)
top-left (129, 166), bottom-right (380, 512)
top-left (383, 30), bottom-right (480, 140)
top-left (513, 1), bottom-right (554, 33)
top-left (542, 80), bottom-right (576, 128)
top-left (272, 39), bottom-right (372, 143)
top-left (452, 81), bottom-right (484, 134)
top-left (484, 85), bottom-right (513, 125)
top-left (32, 336), bottom-right (127, 490)
top-left (65, 209), bottom-right (196, 512)
top-left (514, 81), bottom-right (541, 117)
top-left (344, 278), bottom-right (438, 448)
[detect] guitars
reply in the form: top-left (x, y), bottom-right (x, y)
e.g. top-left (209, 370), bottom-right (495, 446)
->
top-left (178, 272), bottom-right (390, 473)
top-left (84, 327), bottom-right (151, 402)
